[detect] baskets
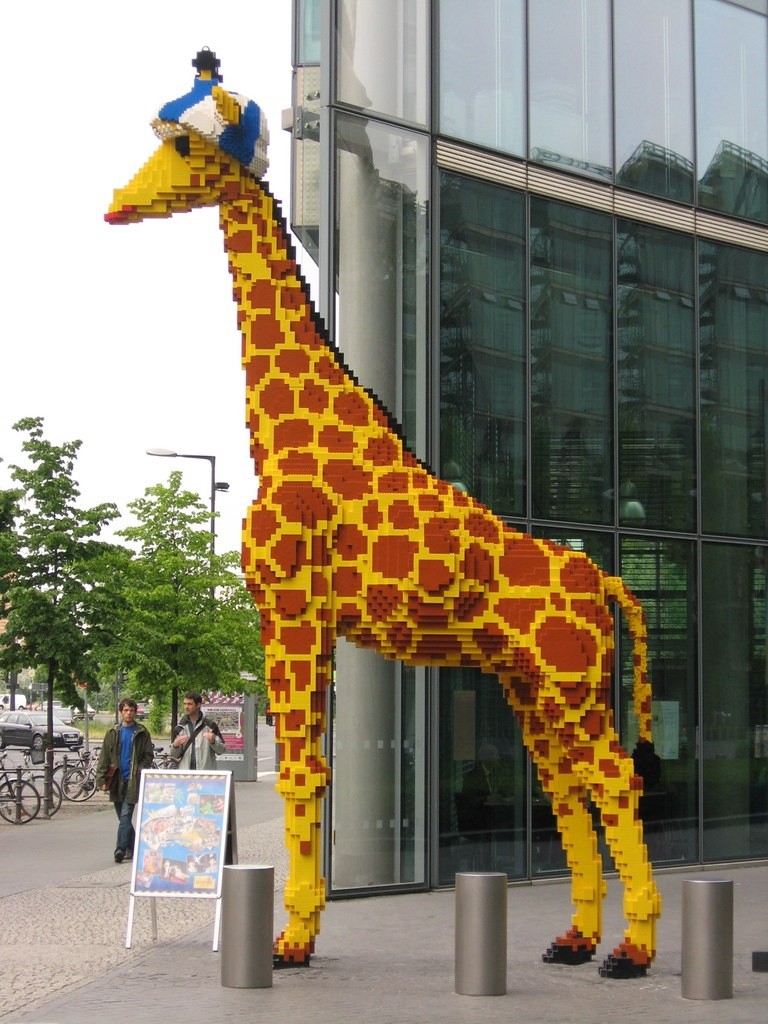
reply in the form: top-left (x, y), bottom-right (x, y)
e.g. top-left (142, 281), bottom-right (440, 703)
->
top-left (30, 747), bottom-right (46, 765)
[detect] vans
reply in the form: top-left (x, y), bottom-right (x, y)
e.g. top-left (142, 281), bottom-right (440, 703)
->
top-left (0, 693), bottom-right (27, 710)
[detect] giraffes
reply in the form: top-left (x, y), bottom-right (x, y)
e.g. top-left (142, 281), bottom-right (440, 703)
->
top-left (102, 46), bottom-right (670, 979)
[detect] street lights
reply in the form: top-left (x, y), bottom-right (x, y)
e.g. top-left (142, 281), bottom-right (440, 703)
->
top-left (144, 446), bottom-right (230, 636)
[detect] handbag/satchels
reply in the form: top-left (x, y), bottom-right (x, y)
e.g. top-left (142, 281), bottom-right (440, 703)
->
top-left (164, 757), bottom-right (178, 770)
top-left (104, 767), bottom-right (119, 791)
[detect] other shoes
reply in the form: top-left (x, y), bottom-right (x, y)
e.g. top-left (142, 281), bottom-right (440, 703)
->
top-left (124, 848), bottom-right (133, 860)
top-left (114, 849), bottom-right (125, 863)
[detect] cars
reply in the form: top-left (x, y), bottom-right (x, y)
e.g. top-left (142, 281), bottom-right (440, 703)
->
top-left (136, 703), bottom-right (152, 719)
top-left (36, 700), bottom-right (96, 725)
top-left (0, 709), bottom-right (85, 752)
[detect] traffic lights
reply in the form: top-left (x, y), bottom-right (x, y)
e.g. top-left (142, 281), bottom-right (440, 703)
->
top-left (120, 669), bottom-right (128, 684)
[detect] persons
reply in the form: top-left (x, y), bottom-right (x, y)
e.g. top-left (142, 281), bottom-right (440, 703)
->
top-left (170, 691), bottom-right (226, 770)
top-left (96, 698), bottom-right (154, 863)
top-left (266, 699), bottom-right (272, 726)
top-left (459, 743), bottom-right (511, 840)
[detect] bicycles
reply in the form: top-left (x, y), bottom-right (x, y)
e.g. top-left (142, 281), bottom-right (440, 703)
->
top-left (0, 751), bottom-right (41, 826)
top-left (0, 743), bottom-right (172, 819)
